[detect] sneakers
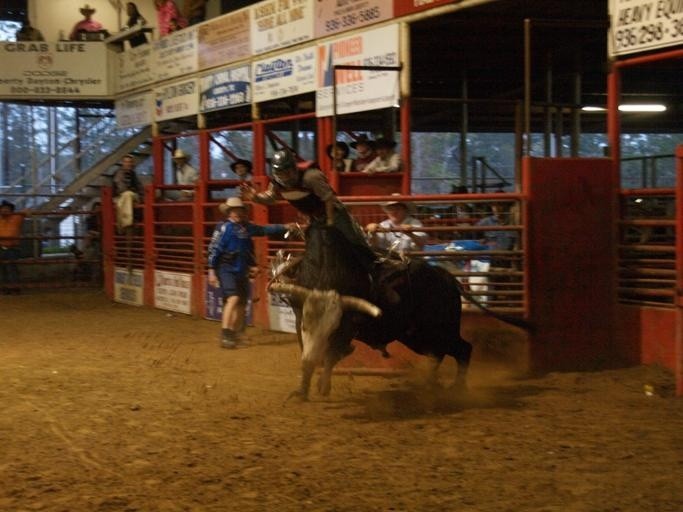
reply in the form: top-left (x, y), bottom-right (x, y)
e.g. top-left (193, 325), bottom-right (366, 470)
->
top-left (219, 335), bottom-right (234, 348)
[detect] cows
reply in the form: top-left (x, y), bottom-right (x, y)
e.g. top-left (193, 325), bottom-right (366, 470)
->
top-left (270, 221), bottom-right (539, 402)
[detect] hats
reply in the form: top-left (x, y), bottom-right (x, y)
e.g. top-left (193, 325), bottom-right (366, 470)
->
top-left (219, 196), bottom-right (251, 217)
top-left (170, 148), bottom-right (193, 164)
top-left (230, 158), bottom-right (253, 175)
top-left (326, 133), bottom-right (397, 161)
top-left (79, 3), bottom-right (97, 16)
top-left (380, 191), bottom-right (417, 216)
top-left (0, 200), bottom-right (15, 209)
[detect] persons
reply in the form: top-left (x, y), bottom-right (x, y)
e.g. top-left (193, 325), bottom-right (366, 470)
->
top-left (473, 188), bottom-right (518, 268)
top-left (120, 3), bottom-right (148, 49)
top-left (431, 184), bottom-right (481, 240)
top-left (69, 4), bottom-right (101, 41)
top-left (152, 0), bottom-right (188, 40)
top-left (0, 199), bottom-right (31, 295)
top-left (183, 1), bottom-right (208, 28)
top-left (364, 192), bottom-right (428, 260)
top-left (86, 133), bottom-right (401, 292)
top-left (240, 150), bottom-right (402, 303)
top-left (15, 20), bottom-right (44, 42)
top-left (206, 196), bottom-right (299, 348)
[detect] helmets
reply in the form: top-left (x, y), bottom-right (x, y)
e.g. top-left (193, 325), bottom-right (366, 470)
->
top-left (270, 148), bottom-right (295, 172)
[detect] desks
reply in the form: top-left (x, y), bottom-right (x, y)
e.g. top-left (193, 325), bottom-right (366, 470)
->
top-left (105, 25), bottom-right (154, 50)
